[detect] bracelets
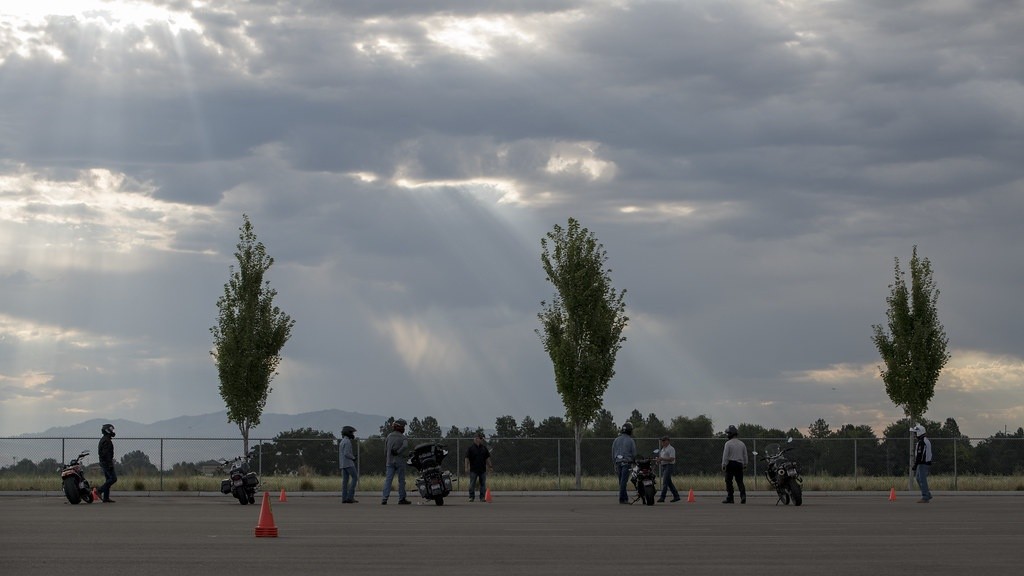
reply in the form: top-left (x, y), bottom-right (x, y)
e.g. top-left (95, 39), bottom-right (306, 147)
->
top-left (490, 466), bottom-right (493, 468)
top-left (662, 458), bottom-right (663, 460)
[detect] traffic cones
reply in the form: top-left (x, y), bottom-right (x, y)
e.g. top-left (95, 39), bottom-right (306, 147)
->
top-left (889, 487), bottom-right (897, 501)
top-left (485, 488), bottom-right (492, 503)
top-left (687, 488), bottom-right (696, 502)
top-left (255, 492), bottom-right (278, 538)
top-left (279, 488), bottom-right (287, 502)
top-left (92, 488), bottom-right (99, 500)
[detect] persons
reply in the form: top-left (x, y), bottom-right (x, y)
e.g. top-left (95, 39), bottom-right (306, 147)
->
top-left (656, 436), bottom-right (680, 502)
top-left (612, 422), bottom-right (637, 504)
top-left (94, 424), bottom-right (117, 503)
top-left (912, 426), bottom-right (933, 503)
top-left (382, 420), bottom-right (411, 505)
top-left (339, 426), bottom-right (358, 504)
top-left (722, 425), bottom-right (748, 504)
top-left (465, 433), bottom-right (493, 502)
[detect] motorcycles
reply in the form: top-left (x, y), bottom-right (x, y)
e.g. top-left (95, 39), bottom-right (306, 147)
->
top-left (407, 440), bottom-right (458, 506)
top-left (219, 454), bottom-right (259, 505)
top-left (57, 449), bottom-right (94, 505)
top-left (752, 437), bottom-right (803, 506)
top-left (615, 449), bottom-right (660, 506)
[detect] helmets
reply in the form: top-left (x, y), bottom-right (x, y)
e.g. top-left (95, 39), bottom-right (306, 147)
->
top-left (341, 426), bottom-right (357, 439)
top-left (622, 422), bottom-right (633, 435)
top-left (394, 418), bottom-right (407, 433)
top-left (725, 425), bottom-right (737, 438)
top-left (909, 426), bottom-right (926, 437)
top-left (102, 424), bottom-right (115, 437)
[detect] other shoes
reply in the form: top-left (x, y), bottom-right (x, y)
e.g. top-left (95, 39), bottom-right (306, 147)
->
top-left (103, 498), bottom-right (115, 503)
top-left (469, 496), bottom-right (474, 502)
top-left (670, 495), bottom-right (680, 502)
top-left (741, 497), bottom-right (746, 504)
top-left (657, 498), bottom-right (664, 502)
top-left (382, 497), bottom-right (388, 505)
top-left (480, 497), bottom-right (486, 502)
top-left (94, 488), bottom-right (102, 500)
top-left (347, 499), bottom-right (359, 504)
top-left (398, 497), bottom-right (411, 504)
top-left (722, 500), bottom-right (734, 503)
top-left (917, 496), bottom-right (933, 503)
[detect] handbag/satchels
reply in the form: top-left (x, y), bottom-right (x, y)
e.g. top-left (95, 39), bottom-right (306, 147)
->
top-left (220, 479), bottom-right (232, 494)
top-left (245, 472), bottom-right (258, 488)
